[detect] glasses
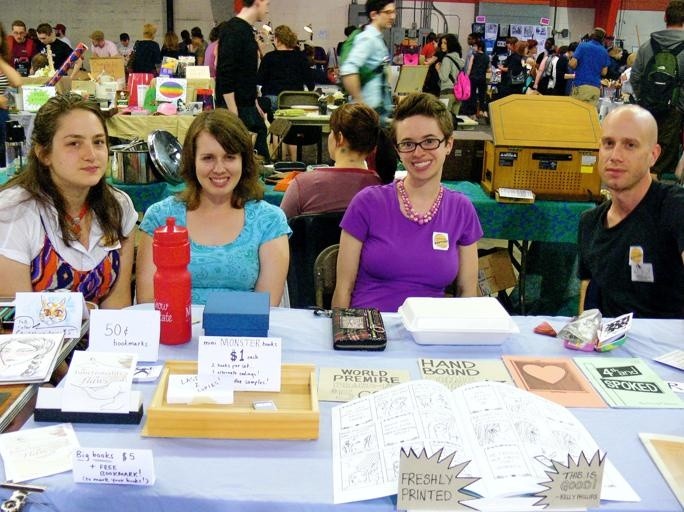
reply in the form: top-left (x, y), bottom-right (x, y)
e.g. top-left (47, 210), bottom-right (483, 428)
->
top-left (392, 133), bottom-right (448, 153)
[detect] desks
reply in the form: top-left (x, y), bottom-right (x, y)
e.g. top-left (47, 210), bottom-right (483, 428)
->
top-left (1, 290), bottom-right (100, 433)
top-left (0, 46), bottom-right (607, 315)
top-left (1, 306), bottom-right (683, 512)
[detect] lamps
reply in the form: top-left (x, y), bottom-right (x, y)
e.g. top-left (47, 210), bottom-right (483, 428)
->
top-left (262, 20), bottom-right (273, 36)
top-left (302, 23), bottom-right (315, 43)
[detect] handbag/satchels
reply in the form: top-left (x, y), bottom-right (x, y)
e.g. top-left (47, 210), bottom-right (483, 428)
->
top-left (338, 29), bottom-right (377, 96)
top-left (511, 72), bottom-right (524, 84)
top-left (331, 308), bottom-right (387, 351)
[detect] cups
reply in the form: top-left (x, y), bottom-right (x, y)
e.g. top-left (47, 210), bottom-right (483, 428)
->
top-left (319, 99), bottom-right (328, 115)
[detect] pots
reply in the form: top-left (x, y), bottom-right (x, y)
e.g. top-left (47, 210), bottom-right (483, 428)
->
top-left (109, 142), bottom-right (159, 184)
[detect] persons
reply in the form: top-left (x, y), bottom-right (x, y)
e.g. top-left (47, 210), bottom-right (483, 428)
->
top-left (576, 103), bottom-right (684, 319)
top-left (134, 108), bottom-right (291, 309)
top-left (1, 20), bottom-right (224, 160)
top-left (329, 93), bottom-right (481, 312)
top-left (213, 0), bottom-right (331, 164)
top-left (2, 93), bottom-right (133, 314)
top-left (631, 1), bottom-right (684, 187)
top-left (336, 1), bottom-right (400, 186)
top-left (420, 26), bottom-right (639, 126)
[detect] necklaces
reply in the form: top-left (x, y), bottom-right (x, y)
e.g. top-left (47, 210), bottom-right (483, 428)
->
top-left (395, 178), bottom-right (445, 224)
top-left (59, 205), bottom-right (89, 233)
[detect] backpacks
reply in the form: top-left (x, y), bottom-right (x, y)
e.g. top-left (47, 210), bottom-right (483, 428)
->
top-left (442, 55), bottom-right (471, 100)
top-left (643, 38), bottom-right (684, 114)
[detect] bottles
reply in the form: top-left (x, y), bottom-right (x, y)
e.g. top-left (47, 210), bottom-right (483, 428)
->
top-left (151, 216), bottom-right (194, 346)
top-left (5, 119), bottom-right (25, 178)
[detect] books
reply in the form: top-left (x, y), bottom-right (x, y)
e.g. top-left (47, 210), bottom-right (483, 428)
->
top-left (496, 189), bottom-right (536, 204)
top-left (328, 376), bottom-right (642, 506)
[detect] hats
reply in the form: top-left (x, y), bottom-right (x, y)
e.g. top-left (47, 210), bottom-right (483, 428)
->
top-left (88, 31), bottom-right (104, 40)
top-left (53, 24), bottom-right (65, 31)
